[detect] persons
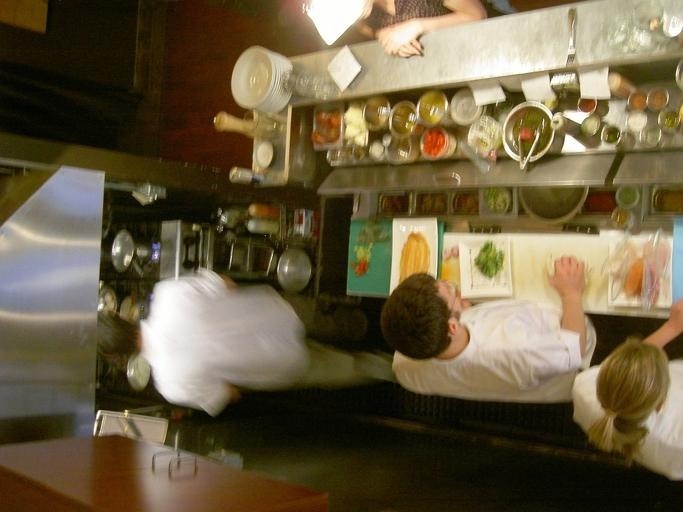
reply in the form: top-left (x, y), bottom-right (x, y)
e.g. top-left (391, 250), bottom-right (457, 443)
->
top-left (379, 254), bottom-right (597, 406)
top-left (352, 0), bottom-right (490, 58)
top-left (571, 297), bottom-right (683, 483)
top-left (98, 265), bottom-right (398, 418)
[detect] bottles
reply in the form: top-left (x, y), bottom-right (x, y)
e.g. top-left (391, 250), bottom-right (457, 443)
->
top-left (247, 204), bottom-right (281, 218)
top-left (244, 218), bottom-right (281, 236)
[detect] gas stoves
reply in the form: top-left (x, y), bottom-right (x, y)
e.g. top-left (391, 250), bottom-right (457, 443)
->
top-left (110, 213), bottom-right (208, 326)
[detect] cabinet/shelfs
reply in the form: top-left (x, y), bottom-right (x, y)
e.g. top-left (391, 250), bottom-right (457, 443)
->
top-left (0, 433), bottom-right (330, 512)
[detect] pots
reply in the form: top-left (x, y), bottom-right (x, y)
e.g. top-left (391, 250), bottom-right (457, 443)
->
top-left (110, 228), bottom-right (151, 280)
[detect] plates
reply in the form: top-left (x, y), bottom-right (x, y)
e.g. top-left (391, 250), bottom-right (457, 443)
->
top-left (230, 45), bottom-right (295, 114)
top-left (606, 231), bottom-right (674, 310)
top-left (253, 139), bottom-right (274, 170)
top-left (388, 215), bottom-right (439, 300)
top-left (457, 235), bottom-right (515, 301)
top-left (125, 351), bottom-right (152, 393)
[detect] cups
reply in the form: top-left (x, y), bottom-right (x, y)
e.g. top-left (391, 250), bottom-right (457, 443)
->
top-left (632, 1), bottom-right (683, 49)
top-left (240, 109), bottom-right (278, 139)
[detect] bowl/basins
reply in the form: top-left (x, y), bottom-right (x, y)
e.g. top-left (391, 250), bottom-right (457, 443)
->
top-left (359, 86), bottom-right (506, 165)
top-left (276, 246), bottom-right (315, 298)
top-left (614, 183), bottom-right (641, 210)
top-left (501, 98), bottom-right (559, 165)
top-left (574, 85), bottom-right (683, 149)
top-left (517, 184), bottom-right (591, 227)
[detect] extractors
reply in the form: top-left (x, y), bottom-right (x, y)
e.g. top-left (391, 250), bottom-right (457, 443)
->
top-left (0, 163), bottom-right (106, 450)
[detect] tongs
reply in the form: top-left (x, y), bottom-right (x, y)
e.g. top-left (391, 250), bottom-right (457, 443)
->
top-left (515, 117), bottom-right (546, 172)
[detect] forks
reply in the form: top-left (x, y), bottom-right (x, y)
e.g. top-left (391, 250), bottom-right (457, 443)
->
top-left (561, 6), bottom-right (579, 70)
